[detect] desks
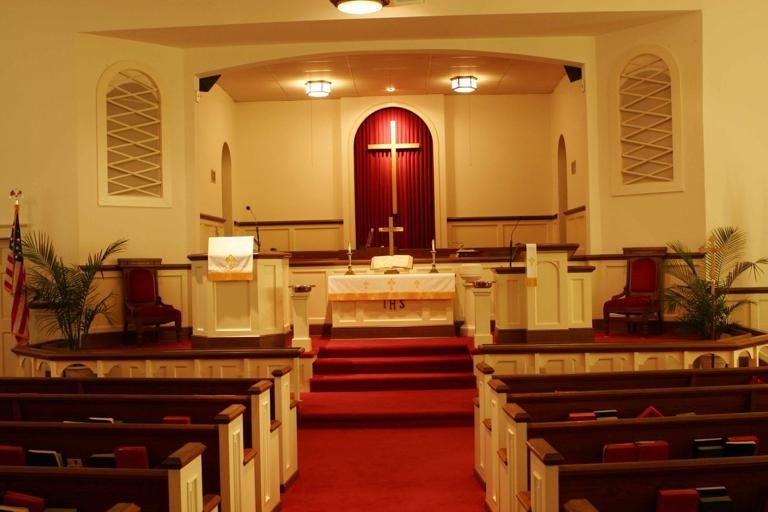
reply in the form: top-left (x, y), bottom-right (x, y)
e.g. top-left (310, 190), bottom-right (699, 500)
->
top-left (327, 273), bottom-right (459, 328)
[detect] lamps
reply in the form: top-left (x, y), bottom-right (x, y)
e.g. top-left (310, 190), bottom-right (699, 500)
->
top-left (449, 75), bottom-right (479, 94)
top-left (305, 80), bottom-right (332, 99)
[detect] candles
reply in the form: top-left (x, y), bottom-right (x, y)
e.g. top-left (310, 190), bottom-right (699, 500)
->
top-left (347, 242), bottom-right (351, 253)
top-left (431, 239), bottom-right (435, 251)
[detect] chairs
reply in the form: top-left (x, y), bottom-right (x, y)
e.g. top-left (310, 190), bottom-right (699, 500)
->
top-left (601, 244), bottom-right (670, 338)
top-left (116, 255), bottom-right (185, 347)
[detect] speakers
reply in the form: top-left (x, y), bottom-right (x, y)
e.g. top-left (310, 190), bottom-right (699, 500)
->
top-left (564, 65), bottom-right (581, 82)
top-left (199, 75), bottom-right (221, 92)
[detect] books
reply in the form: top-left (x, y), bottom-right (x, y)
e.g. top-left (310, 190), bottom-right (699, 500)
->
top-left (566, 406), bottom-right (761, 511)
top-left (371, 254), bottom-right (414, 270)
top-left (0, 413), bottom-right (191, 512)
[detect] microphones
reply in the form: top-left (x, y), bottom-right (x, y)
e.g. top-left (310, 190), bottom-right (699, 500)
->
top-left (510, 216), bottom-right (521, 266)
top-left (246, 205), bottom-right (260, 252)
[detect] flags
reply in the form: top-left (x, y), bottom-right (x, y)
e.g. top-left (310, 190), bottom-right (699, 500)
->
top-left (2, 204), bottom-right (32, 348)
